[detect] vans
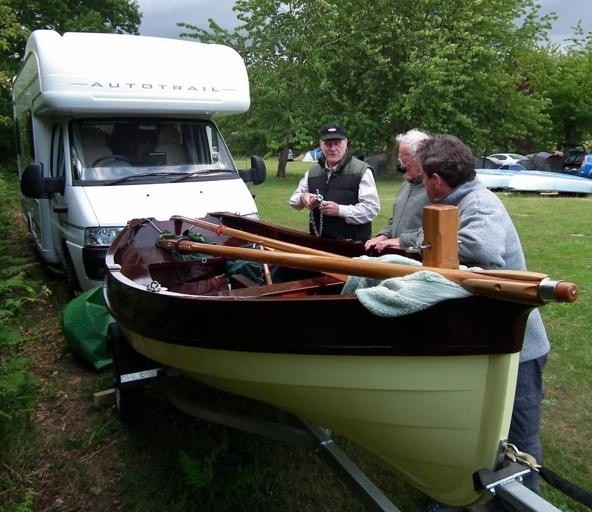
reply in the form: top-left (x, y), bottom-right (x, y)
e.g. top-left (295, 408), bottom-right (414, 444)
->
top-left (277, 149), bottom-right (294, 162)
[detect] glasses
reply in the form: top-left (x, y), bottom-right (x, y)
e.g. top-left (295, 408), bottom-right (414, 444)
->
top-left (397, 155), bottom-right (416, 166)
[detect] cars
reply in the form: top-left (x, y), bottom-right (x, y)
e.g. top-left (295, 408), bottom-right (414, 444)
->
top-left (489, 153), bottom-right (529, 167)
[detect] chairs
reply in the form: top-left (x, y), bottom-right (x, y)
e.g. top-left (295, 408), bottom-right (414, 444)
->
top-left (79, 129), bottom-right (114, 166)
top-left (151, 124), bottom-right (187, 164)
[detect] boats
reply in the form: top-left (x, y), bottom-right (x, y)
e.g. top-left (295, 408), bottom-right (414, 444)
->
top-left (475, 164), bottom-right (592, 197)
top-left (101, 207), bottom-right (550, 506)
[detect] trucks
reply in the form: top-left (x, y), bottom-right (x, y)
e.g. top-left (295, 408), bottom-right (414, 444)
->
top-left (7, 27), bottom-right (271, 306)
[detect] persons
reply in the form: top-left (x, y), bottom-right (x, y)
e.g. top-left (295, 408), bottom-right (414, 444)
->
top-left (289, 124), bottom-right (381, 245)
top-left (363, 127), bottom-right (433, 252)
top-left (401, 132), bottom-right (555, 491)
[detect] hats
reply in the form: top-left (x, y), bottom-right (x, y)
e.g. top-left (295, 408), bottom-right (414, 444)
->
top-left (318, 123), bottom-right (345, 140)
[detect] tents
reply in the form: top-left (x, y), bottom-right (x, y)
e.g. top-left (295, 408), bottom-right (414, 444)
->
top-left (301, 147), bottom-right (325, 164)
top-left (517, 151), bottom-right (565, 174)
top-left (365, 153), bottom-right (406, 178)
top-left (473, 156), bottom-right (504, 169)
